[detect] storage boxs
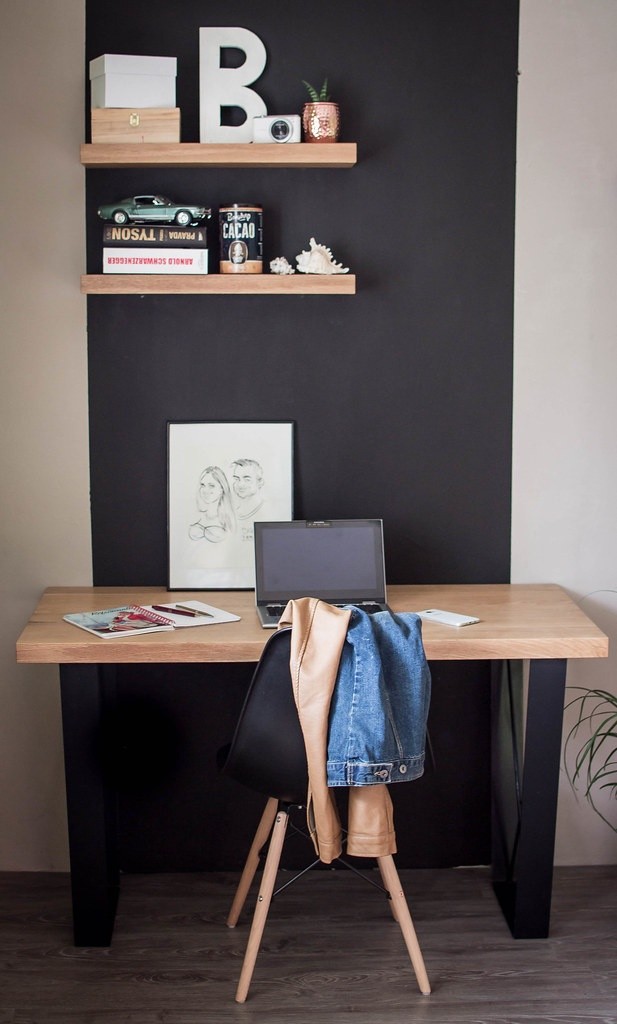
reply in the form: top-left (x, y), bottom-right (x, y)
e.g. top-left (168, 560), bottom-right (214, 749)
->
top-left (88, 53), bottom-right (178, 108)
top-left (90, 108), bottom-right (181, 142)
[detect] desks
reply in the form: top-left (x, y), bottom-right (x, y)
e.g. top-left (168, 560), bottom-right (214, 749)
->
top-left (16, 584), bottom-right (610, 950)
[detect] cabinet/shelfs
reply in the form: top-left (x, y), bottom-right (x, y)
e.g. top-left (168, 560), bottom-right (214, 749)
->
top-left (80, 141), bottom-right (357, 293)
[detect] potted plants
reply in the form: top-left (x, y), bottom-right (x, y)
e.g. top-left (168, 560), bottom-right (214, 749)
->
top-left (299, 79), bottom-right (343, 142)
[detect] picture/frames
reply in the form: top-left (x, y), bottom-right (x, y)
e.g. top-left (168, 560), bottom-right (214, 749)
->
top-left (166, 418), bottom-right (300, 593)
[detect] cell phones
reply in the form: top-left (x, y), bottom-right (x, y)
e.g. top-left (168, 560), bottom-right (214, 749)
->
top-left (416, 608), bottom-right (480, 627)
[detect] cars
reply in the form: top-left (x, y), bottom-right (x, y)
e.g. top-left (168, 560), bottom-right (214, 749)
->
top-left (97, 193), bottom-right (213, 226)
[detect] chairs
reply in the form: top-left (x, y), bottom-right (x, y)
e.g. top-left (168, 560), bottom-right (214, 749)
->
top-left (226, 622), bottom-right (433, 1003)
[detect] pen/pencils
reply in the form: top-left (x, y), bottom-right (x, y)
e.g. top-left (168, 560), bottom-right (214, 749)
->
top-left (176, 605), bottom-right (214, 618)
top-left (151, 604), bottom-right (196, 617)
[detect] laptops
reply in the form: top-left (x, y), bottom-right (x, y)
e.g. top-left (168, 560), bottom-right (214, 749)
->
top-left (253, 518), bottom-right (389, 629)
